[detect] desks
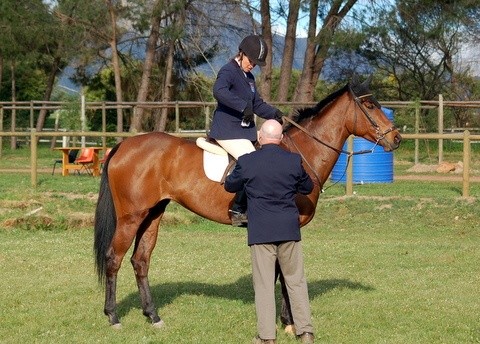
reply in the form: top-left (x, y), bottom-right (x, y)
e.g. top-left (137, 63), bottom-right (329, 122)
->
top-left (52, 147), bottom-right (107, 177)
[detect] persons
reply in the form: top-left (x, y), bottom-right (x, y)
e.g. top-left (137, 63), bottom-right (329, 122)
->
top-left (223, 118), bottom-right (318, 343)
top-left (208, 34), bottom-right (288, 222)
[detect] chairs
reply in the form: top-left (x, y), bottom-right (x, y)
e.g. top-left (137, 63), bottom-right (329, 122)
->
top-left (74, 148), bottom-right (94, 177)
top-left (99, 148), bottom-right (112, 174)
top-left (52, 149), bottom-right (79, 176)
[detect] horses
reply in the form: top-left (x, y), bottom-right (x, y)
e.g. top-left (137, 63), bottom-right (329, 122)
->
top-left (93, 72), bottom-right (402, 340)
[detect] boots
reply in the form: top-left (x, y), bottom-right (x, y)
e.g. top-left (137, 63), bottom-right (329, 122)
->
top-left (227, 189), bottom-right (249, 226)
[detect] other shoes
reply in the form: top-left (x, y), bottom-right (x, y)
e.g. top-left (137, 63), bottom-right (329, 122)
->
top-left (301, 332), bottom-right (313, 344)
top-left (256, 335), bottom-right (274, 344)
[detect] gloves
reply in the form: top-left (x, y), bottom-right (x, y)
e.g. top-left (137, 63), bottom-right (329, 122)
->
top-left (241, 106), bottom-right (254, 124)
top-left (274, 109), bottom-right (284, 125)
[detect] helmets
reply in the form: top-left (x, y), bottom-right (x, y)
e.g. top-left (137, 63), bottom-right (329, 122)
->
top-left (240, 35), bottom-right (268, 66)
